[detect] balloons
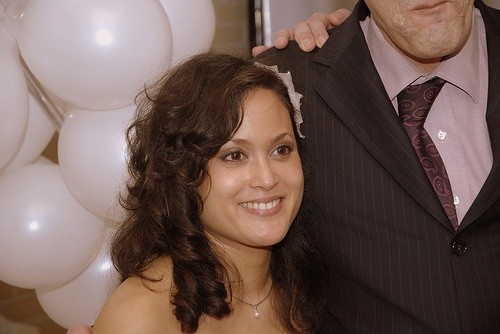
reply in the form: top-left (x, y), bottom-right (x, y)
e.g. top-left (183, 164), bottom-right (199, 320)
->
top-left (0, 0), bottom-right (79, 173)
top-left (57, 99), bottom-right (153, 222)
top-left (0, 163), bottom-right (107, 289)
top-left (159, 1), bottom-right (215, 64)
top-left (36, 224), bottom-right (124, 330)
top-left (16, 1), bottom-right (173, 109)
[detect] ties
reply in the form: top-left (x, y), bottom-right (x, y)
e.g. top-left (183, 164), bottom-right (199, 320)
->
top-left (397, 77), bottom-right (459, 231)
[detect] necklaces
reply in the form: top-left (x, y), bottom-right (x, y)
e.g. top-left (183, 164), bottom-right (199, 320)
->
top-left (225, 278), bottom-right (272, 317)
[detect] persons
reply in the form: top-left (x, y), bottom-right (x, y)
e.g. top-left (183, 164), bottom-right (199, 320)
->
top-left (252, 8), bottom-right (351, 58)
top-left (92, 53), bottom-right (327, 334)
top-left (68, 0), bottom-right (500, 334)
